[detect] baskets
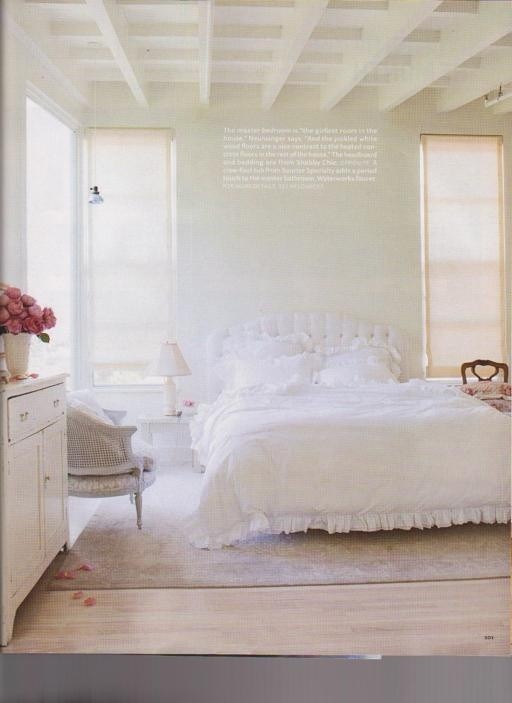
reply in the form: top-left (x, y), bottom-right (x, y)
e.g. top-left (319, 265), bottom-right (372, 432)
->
top-left (3, 331), bottom-right (31, 374)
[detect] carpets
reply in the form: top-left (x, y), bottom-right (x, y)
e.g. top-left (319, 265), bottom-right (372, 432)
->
top-left (47, 463), bottom-right (511, 595)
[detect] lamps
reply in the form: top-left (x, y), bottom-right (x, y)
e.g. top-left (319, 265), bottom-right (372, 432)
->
top-left (148, 339), bottom-right (193, 415)
top-left (87, 186), bottom-right (103, 203)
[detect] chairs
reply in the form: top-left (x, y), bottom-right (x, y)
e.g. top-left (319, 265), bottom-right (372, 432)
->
top-left (461, 359), bottom-right (508, 386)
top-left (67, 389), bottom-right (158, 531)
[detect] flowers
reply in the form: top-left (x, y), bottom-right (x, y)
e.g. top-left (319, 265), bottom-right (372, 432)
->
top-left (0, 282), bottom-right (57, 344)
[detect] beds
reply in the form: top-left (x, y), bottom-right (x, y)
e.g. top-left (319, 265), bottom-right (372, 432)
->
top-left (200, 312), bottom-right (511, 539)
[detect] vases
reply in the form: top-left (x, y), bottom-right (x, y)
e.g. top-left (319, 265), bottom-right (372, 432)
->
top-left (2, 332), bottom-right (32, 379)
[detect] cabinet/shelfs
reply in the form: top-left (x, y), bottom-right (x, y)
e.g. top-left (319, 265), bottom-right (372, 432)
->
top-left (0, 373), bottom-right (71, 649)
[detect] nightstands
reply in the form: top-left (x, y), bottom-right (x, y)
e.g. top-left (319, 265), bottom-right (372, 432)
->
top-left (138, 410), bottom-right (200, 470)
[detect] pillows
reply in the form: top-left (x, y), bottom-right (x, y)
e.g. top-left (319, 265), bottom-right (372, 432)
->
top-left (223, 331), bottom-right (403, 397)
